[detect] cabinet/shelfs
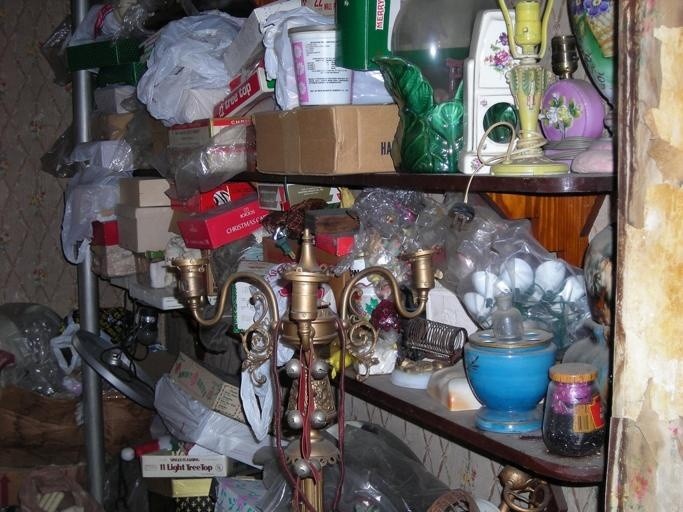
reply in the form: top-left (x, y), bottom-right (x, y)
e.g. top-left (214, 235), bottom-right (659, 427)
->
top-left (75, 0), bottom-right (682, 512)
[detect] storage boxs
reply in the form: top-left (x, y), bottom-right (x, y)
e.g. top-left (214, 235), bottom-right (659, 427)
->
top-left (179, 197), bottom-right (270, 248)
top-left (92, 220), bottom-right (119, 245)
top-left (115, 205), bottom-right (173, 253)
top-left (172, 181), bottom-right (254, 213)
top-left (154, 371), bottom-right (289, 472)
top-left (258, 183), bottom-right (341, 211)
top-left (119, 176), bottom-right (169, 206)
top-left (255, 104), bottom-right (404, 175)
top-left (172, 353), bottom-right (302, 442)
top-left (90, 245), bottom-right (140, 278)
top-left (148, 478), bottom-right (258, 511)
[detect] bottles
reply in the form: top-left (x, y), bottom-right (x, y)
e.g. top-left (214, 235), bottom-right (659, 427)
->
top-left (543, 363), bottom-right (606, 457)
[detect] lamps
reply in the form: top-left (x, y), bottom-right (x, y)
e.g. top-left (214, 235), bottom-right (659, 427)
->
top-left (174, 229), bottom-right (434, 512)
